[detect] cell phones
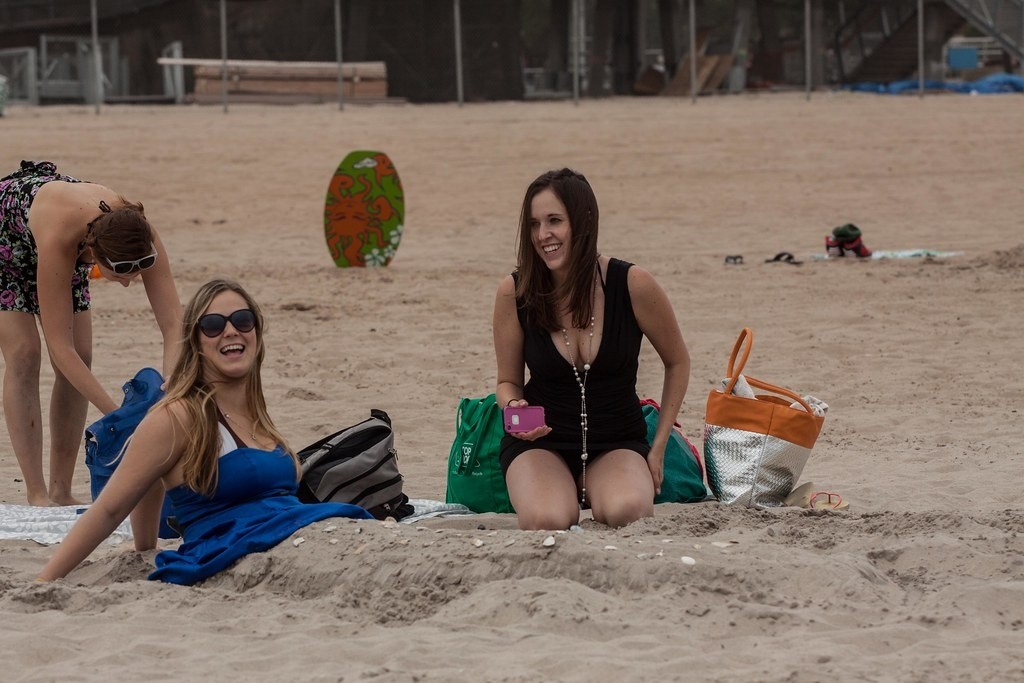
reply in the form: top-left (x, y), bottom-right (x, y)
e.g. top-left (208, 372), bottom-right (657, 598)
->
top-left (502, 406), bottom-right (544, 434)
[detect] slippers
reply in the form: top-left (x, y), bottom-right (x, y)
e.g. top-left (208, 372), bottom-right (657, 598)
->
top-left (724, 255), bottom-right (742, 265)
top-left (765, 252), bottom-right (803, 264)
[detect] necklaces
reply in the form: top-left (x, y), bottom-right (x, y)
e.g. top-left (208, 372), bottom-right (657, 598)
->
top-left (219, 403), bottom-right (258, 441)
top-left (555, 255), bottom-right (598, 503)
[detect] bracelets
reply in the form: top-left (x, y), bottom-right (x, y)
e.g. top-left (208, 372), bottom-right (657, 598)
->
top-left (505, 399), bottom-right (519, 407)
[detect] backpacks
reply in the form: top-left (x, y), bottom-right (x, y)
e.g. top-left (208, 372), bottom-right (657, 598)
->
top-left (296, 406), bottom-right (414, 522)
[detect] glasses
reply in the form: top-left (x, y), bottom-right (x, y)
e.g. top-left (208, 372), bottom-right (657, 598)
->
top-left (199, 307), bottom-right (257, 338)
top-left (101, 241), bottom-right (159, 275)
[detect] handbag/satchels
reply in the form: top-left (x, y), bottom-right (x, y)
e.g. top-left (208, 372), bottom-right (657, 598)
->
top-left (74, 368), bottom-right (166, 513)
top-left (704, 327), bottom-right (829, 508)
top-left (641, 398), bottom-right (706, 502)
top-left (445, 393), bottom-right (514, 513)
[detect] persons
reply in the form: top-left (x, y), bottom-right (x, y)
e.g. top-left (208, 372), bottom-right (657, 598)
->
top-left (37, 280), bottom-right (376, 593)
top-left (0, 160), bottom-right (183, 508)
top-left (492, 168), bottom-right (690, 531)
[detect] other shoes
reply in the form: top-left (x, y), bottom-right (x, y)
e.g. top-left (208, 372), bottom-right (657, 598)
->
top-left (825, 234), bottom-right (872, 260)
